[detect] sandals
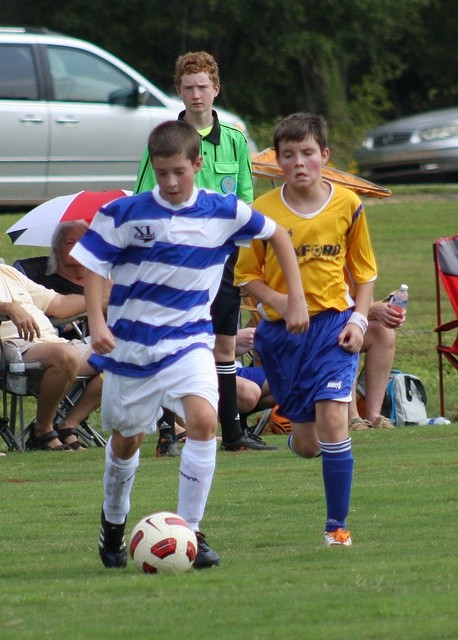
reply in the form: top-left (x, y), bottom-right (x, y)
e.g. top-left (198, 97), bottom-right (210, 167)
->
top-left (53, 423), bottom-right (86, 450)
top-left (24, 422), bottom-right (72, 452)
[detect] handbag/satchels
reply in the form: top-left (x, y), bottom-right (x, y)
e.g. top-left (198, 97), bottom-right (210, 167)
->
top-left (357, 365), bottom-right (427, 426)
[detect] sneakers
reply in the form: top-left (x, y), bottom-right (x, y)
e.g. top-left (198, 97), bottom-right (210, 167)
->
top-left (219, 427), bottom-right (277, 450)
top-left (156, 432), bottom-right (179, 457)
top-left (347, 417), bottom-right (367, 431)
top-left (98, 499), bottom-right (128, 569)
top-left (367, 415), bottom-right (394, 429)
top-left (323, 528), bottom-right (351, 546)
top-left (191, 532), bottom-right (220, 570)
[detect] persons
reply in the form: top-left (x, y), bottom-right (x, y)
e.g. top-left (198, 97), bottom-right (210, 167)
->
top-left (231, 111), bottom-right (377, 553)
top-left (0, 259), bottom-right (114, 452)
top-left (66, 122), bottom-right (311, 569)
top-left (230, 325), bottom-right (276, 420)
top-left (132, 50), bottom-right (278, 454)
top-left (342, 267), bottom-right (407, 431)
top-left (40, 220), bottom-right (89, 339)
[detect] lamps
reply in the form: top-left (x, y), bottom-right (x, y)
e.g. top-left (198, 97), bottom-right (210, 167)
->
top-left (0, 282), bottom-right (94, 454)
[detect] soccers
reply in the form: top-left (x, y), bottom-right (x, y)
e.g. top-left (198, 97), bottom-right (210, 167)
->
top-left (129, 511), bottom-right (197, 574)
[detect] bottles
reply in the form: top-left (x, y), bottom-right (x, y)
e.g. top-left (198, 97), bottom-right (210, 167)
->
top-left (9, 348), bottom-right (26, 376)
top-left (387, 284), bottom-right (409, 321)
top-left (427, 417), bottom-right (451, 425)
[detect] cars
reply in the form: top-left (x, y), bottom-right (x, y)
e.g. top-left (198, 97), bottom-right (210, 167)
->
top-left (354, 107), bottom-right (457, 179)
top-left (0, 28), bottom-right (257, 206)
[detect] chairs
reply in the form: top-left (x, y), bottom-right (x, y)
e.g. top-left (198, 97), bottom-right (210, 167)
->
top-left (18, 258), bottom-right (111, 446)
top-left (433, 237), bottom-right (457, 420)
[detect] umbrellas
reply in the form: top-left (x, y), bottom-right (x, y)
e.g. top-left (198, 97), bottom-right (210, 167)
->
top-left (5, 189), bottom-right (134, 248)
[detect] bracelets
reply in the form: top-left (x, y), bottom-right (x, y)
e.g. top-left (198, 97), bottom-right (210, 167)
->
top-left (345, 309), bottom-right (370, 337)
